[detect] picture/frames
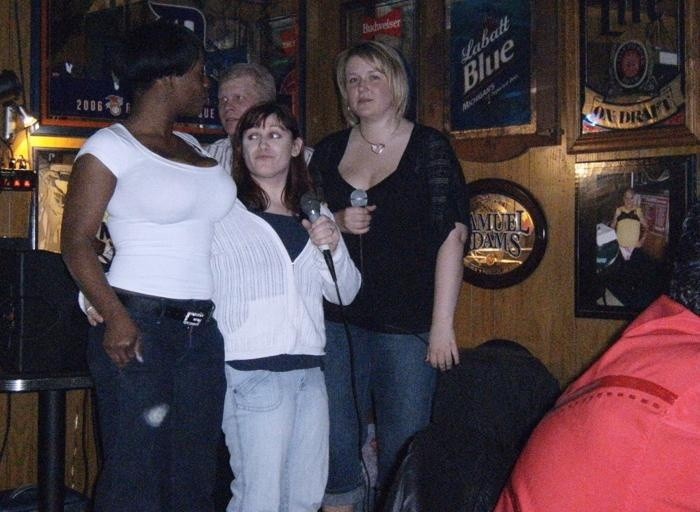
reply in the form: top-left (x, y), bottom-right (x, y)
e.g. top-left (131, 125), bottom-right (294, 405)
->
top-left (573, 157), bottom-right (699, 321)
top-left (563, 2), bottom-right (698, 152)
top-left (30, 1), bottom-right (252, 143)
top-left (255, 15), bottom-right (305, 141)
top-left (458, 176), bottom-right (547, 290)
top-left (32, 147), bottom-right (83, 253)
top-left (434, 1), bottom-right (558, 160)
top-left (345, 5), bottom-right (426, 130)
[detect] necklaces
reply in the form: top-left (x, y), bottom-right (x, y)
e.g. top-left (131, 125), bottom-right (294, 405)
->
top-left (358, 119), bottom-right (403, 155)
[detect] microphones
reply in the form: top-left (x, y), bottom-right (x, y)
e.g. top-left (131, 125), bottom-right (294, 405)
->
top-left (350, 189), bottom-right (368, 258)
top-left (300, 190), bottom-right (337, 282)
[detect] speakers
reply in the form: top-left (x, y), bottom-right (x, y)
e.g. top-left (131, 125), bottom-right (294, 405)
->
top-left (0, 249), bottom-right (91, 380)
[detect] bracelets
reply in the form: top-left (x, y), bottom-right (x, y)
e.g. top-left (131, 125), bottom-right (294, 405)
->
top-left (642, 237), bottom-right (645, 240)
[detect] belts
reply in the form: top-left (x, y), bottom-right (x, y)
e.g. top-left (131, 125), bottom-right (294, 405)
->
top-left (117, 292), bottom-right (215, 327)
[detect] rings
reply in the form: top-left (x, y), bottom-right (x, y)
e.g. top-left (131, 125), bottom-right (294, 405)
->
top-left (86, 306), bottom-right (92, 311)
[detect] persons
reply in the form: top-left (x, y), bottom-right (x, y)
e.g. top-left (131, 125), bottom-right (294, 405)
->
top-left (610, 188), bottom-right (649, 250)
top-left (58, 18), bottom-right (239, 511)
top-left (75, 101), bottom-right (364, 512)
top-left (197, 62), bottom-right (318, 196)
top-left (0, 68), bottom-right (26, 169)
top-left (296, 38), bottom-right (478, 511)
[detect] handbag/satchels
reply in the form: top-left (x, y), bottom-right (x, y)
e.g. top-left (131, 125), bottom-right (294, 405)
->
top-left (383, 338), bottom-right (562, 511)
